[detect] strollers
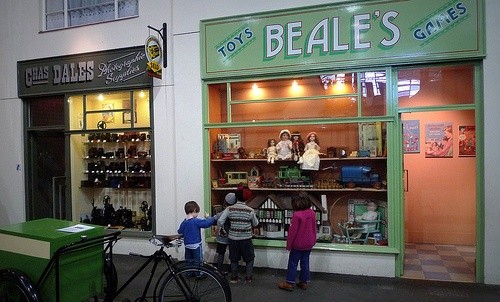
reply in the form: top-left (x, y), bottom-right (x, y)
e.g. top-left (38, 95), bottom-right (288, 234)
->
top-left (333, 211), bottom-right (383, 245)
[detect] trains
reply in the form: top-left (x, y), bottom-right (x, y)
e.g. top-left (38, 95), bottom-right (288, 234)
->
top-left (274, 166), bottom-right (313, 187)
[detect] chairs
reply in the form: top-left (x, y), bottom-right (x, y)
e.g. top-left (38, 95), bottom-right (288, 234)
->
top-left (334, 211), bottom-right (382, 245)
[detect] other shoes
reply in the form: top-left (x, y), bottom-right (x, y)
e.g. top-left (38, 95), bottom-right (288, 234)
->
top-left (230, 277), bottom-right (240, 283)
top-left (278, 282), bottom-right (294, 291)
top-left (245, 280), bottom-right (255, 285)
top-left (296, 281), bottom-right (308, 289)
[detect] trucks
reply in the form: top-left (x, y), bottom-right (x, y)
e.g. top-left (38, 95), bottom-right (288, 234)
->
top-left (337, 165), bottom-right (382, 189)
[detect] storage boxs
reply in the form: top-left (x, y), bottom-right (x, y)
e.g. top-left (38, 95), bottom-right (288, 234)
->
top-left (0, 218), bottom-right (106, 302)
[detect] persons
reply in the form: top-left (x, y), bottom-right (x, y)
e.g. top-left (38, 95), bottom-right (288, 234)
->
top-left (296, 132), bottom-right (320, 170)
top-left (349, 200), bottom-right (378, 239)
top-left (277, 190), bottom-right (317, 291)
top-left (275, 129), bottom-right (292, 161)
top-left (177, 201), bottom-right (217, 280)
top-left (204, 193), bottom-right (238, 277)
top-left (217, 186), bottom-right (260, 284)
top-left (290, 132), bottom-right (305, 162)
top-left (266, 138), bottom-right (277, 164)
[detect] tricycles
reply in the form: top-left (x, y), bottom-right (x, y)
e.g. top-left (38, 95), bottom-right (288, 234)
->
top-left (1, 216), bottom-right (233, 301)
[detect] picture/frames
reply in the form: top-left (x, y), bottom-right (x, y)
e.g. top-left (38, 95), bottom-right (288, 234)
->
top-left (457, 124), bottom-right (476, 156)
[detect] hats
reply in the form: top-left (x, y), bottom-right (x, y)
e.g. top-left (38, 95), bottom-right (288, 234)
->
top-left (225, 193), bottom-right (236, 204)
top-left (235, 186), bottom-right (252, 201)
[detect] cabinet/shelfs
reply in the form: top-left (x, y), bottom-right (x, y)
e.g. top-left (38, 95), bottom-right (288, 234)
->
top-left (79, 140), bottom-right (152, 231)
top-left (211, 156), bottom-right (388, 246)
